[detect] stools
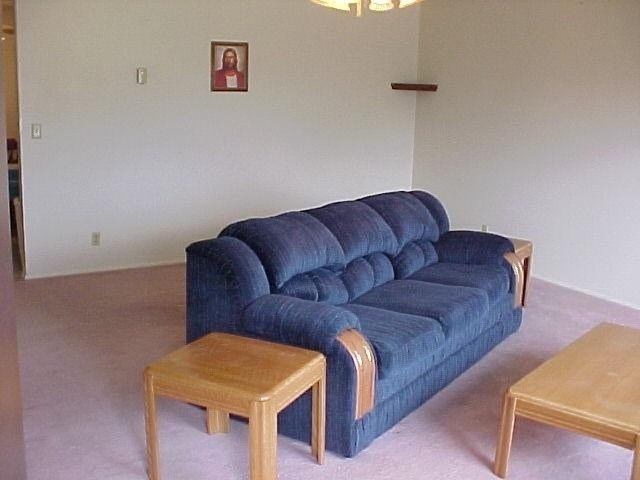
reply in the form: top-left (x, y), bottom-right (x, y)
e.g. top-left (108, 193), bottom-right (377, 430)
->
top-left (144, 332), bottom-right (330, 480)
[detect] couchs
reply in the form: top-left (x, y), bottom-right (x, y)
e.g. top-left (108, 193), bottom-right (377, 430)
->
top-left (187, 190), bottom-right (526, 460)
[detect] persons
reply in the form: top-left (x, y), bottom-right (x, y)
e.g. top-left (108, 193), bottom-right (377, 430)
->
top-left (213, 48), bottom-right (244, 88)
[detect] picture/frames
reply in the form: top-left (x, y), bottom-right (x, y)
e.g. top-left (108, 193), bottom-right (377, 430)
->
top-left (210, 41), bottom-right (250, 93)
